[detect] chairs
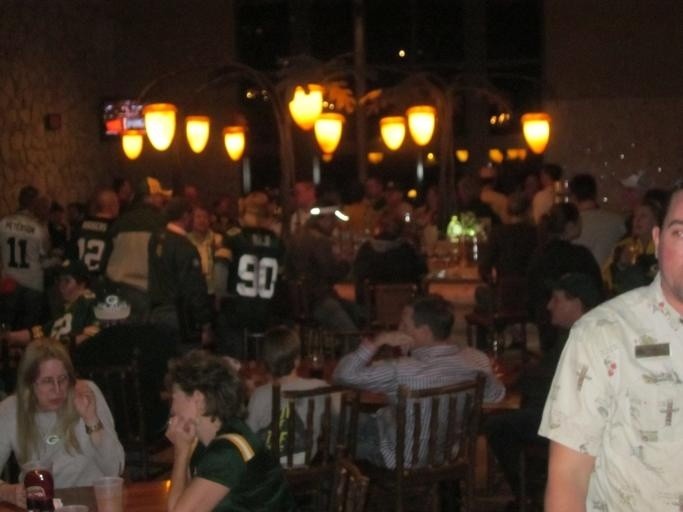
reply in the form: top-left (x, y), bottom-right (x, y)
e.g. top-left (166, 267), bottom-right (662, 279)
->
top-left (0, 209), bottom-right (546, 511)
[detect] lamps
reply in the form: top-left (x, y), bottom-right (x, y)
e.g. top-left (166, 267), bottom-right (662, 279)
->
top-left (120, 122), bottom-right (146, 161)
top-left (405, 105), bottom-right (438, 147)
top-left (222, 125), bottom-right (246, 162)
top-left (142, 103), bottom-right (178, 150)
top-left (315, 113), bottom-right (346, 156)
top-left (378, 114), bottom-right (407, 152)
top-left (286, 82), bottom-right (326, 131)
top-left (519, 112), bottom-right (552, 153)
top-left (184, 114), bottom-right (211, 154)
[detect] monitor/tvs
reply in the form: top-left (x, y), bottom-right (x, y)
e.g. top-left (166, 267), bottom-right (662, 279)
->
top-left (99, 94), bottom-right (151, 143)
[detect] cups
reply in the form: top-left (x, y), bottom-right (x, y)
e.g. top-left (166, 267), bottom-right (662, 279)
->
top-left (92, 476), bottom-right (124, 512)
top-left (22, 460), bottom-right (54, 480)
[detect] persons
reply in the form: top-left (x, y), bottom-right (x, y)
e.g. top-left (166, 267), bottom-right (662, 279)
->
top-left (0, 157), bottom-right (682, 512)
